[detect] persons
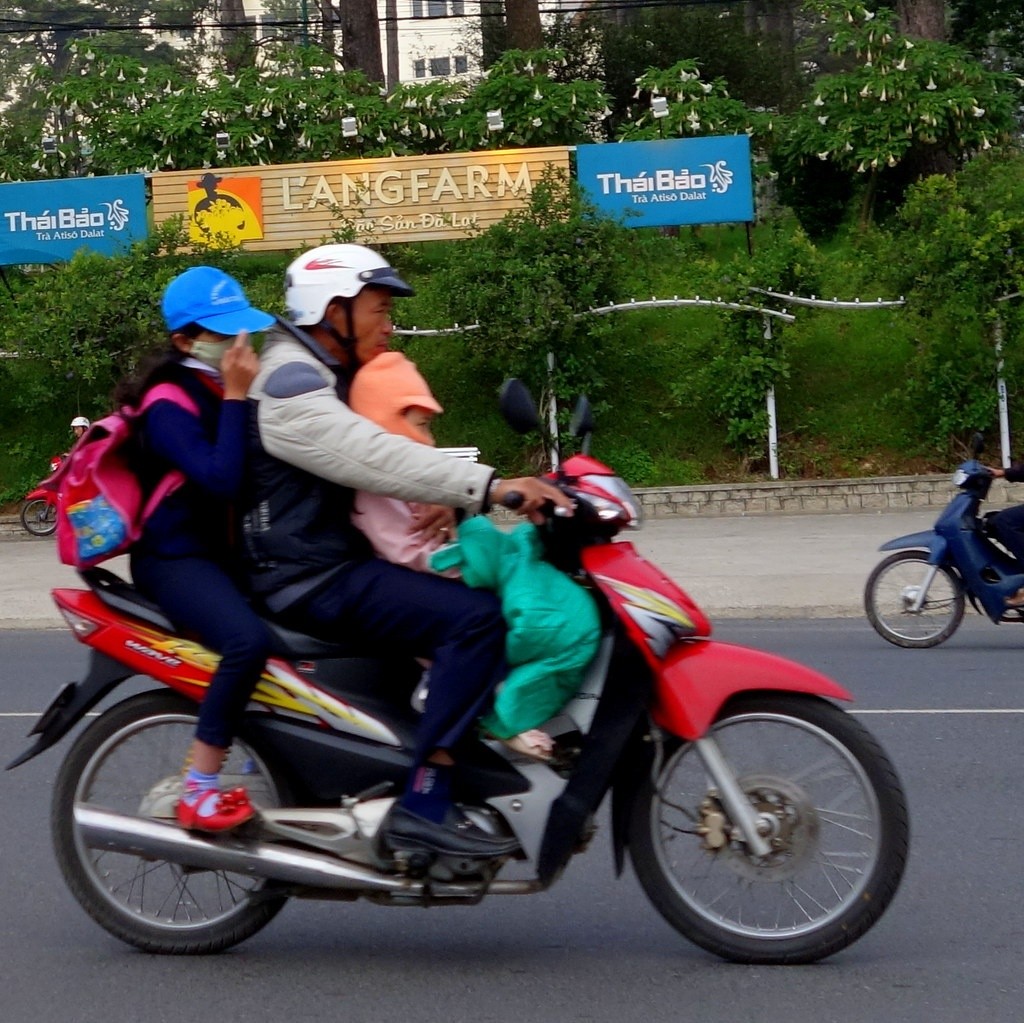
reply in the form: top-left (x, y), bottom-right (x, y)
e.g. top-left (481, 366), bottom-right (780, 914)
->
top-left (351, 351), bottom-right (601, 759)
top-left (237, 243), bottom-right (574, 856)
top-left (982, 465), bottom-right (1024, 608)
top-left (127, 265), bottom-right (276, 832)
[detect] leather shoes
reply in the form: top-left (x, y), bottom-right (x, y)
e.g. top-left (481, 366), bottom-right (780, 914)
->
top-left (173, 786), bottom-right (257, 834)
top-left (382, 804), bottom-right (521, 861)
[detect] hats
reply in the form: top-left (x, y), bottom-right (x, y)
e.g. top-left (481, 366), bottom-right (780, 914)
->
top-left (161, 265), bottom-right (277, 336)
top-left (349, 350), bottom-right (443, 447)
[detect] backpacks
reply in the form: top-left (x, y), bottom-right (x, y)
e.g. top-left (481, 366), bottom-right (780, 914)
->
top-left (55, 383), bottom-right (198, 569)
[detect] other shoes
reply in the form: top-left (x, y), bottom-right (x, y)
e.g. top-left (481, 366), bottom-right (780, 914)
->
top-left (481, 720), bottom-right (555, 763)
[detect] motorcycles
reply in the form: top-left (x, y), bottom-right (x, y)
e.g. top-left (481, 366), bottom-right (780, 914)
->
top-left (864, 433), bottom-right (1024, 648)
top-left (19, 452), bottom-right (69, 536)
top-left (4, 380), bottom-right (909, 965)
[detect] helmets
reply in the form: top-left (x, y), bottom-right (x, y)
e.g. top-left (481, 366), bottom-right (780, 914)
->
top-left (281, 242), bottom-right (409, 327)
top-left (69, 416), bottom-right (91, 430)
